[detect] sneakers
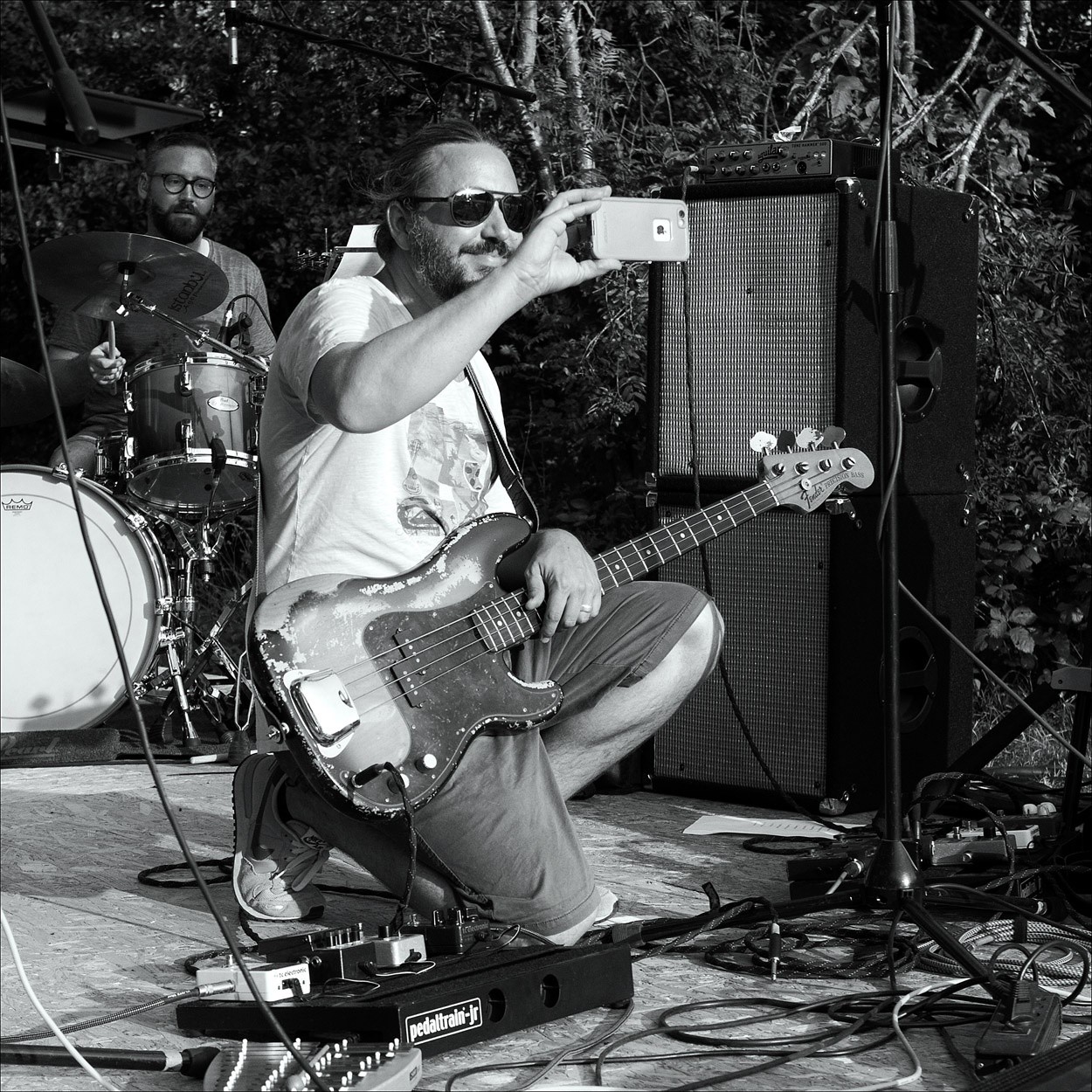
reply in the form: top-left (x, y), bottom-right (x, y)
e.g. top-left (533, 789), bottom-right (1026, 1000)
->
top-left (232, 753), bottom-right (325, 922)
top-left (593, 885), bottom-right (619, 924)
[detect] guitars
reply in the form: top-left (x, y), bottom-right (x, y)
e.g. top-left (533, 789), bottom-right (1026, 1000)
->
top-left (247, 426), bottom-right (880, 824)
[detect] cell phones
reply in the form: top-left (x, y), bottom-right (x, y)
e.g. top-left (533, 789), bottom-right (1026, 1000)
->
top-left (590, 195), bottom-right (690, 262)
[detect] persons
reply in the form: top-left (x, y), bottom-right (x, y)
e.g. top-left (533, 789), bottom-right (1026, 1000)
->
top-left (232, 118), bottom-right (724, 946)
top-left (43, 128), bottom-right (278, 478)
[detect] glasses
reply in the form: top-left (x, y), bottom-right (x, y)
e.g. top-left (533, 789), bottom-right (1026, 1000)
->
top-left (387, 189), bottom-right (535, 233)
top-left (146, 174), bottom-right (217, 198)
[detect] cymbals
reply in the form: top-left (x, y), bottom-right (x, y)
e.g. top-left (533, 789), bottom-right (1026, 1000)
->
top-left (23, 229), bottom-right (229, 323)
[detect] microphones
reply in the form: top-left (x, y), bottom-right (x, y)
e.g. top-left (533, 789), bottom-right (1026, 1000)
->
top-left (226, 0), bottom-right (239, 66)
top-left (213, 301), bottom-right (235, 354)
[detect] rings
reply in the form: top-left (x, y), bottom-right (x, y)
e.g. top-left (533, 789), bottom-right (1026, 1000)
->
top-left (580, 604), bottom-right (593, 612)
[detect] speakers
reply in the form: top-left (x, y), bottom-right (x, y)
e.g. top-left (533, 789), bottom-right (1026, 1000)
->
top-left (643, 175), bottom-right (981, 818)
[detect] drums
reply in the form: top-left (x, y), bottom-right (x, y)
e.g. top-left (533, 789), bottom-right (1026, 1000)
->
top-left (119, 350), bottom-right (262, 515)
top-left (1, 464), bottom-right (170, 734)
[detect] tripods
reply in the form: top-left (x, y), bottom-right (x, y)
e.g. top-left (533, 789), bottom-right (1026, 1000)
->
top-left (141, 512), bottom-right (241, 745)
top-left (613, 1), bottom-right (1092, 999)
